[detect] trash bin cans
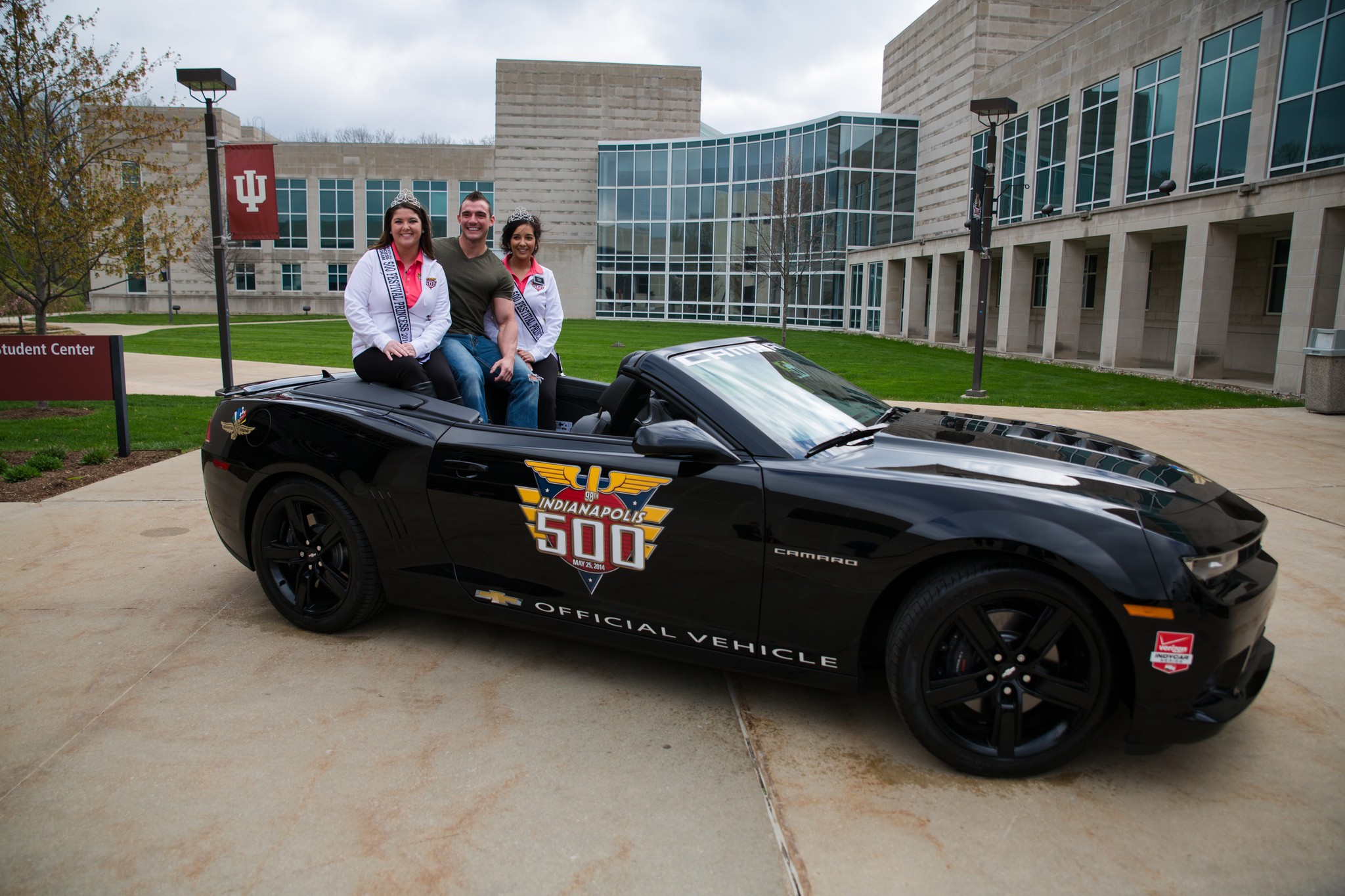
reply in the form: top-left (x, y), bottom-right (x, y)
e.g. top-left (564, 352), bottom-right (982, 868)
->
top-left (1302, 327), bottom-right (1345, 414)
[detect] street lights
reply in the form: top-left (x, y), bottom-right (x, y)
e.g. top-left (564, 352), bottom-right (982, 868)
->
top-left (175, 67), bottom-right (239, 394)
top-left (962, 96), bottom-right (1020, 400)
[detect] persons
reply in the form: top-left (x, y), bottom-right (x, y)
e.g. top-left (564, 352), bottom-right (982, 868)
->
top-left (499, 206), bottom-right (566, 431)
top-left (430, 191), bottom-right (543, 429)
top-left (343, 188), bottom-right (465, 408)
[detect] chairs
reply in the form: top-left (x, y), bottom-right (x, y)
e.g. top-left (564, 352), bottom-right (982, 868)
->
top-left (570, 350), bottom-right (673, 437)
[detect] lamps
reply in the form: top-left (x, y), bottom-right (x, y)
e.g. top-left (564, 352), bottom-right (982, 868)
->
top-left (173, 305), bottom-right (180, 310)
top-left (1159, 179), bottom-right (1177, 196)
top-left (303, 306), bottom-right (311, 311)
top-left (964, 219), bottom-right (971, 229)
top-left (1041, 204), bottom-right (1053, 217)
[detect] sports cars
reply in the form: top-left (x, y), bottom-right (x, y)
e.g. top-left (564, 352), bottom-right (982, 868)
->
top-left (200, 335), bottom-right (1278, 782)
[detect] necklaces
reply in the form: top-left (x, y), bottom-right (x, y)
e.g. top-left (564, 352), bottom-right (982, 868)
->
top-left (405, 269), bottom-right (407, 274)
top-left (510, 259), bottom-right (531, 282)
